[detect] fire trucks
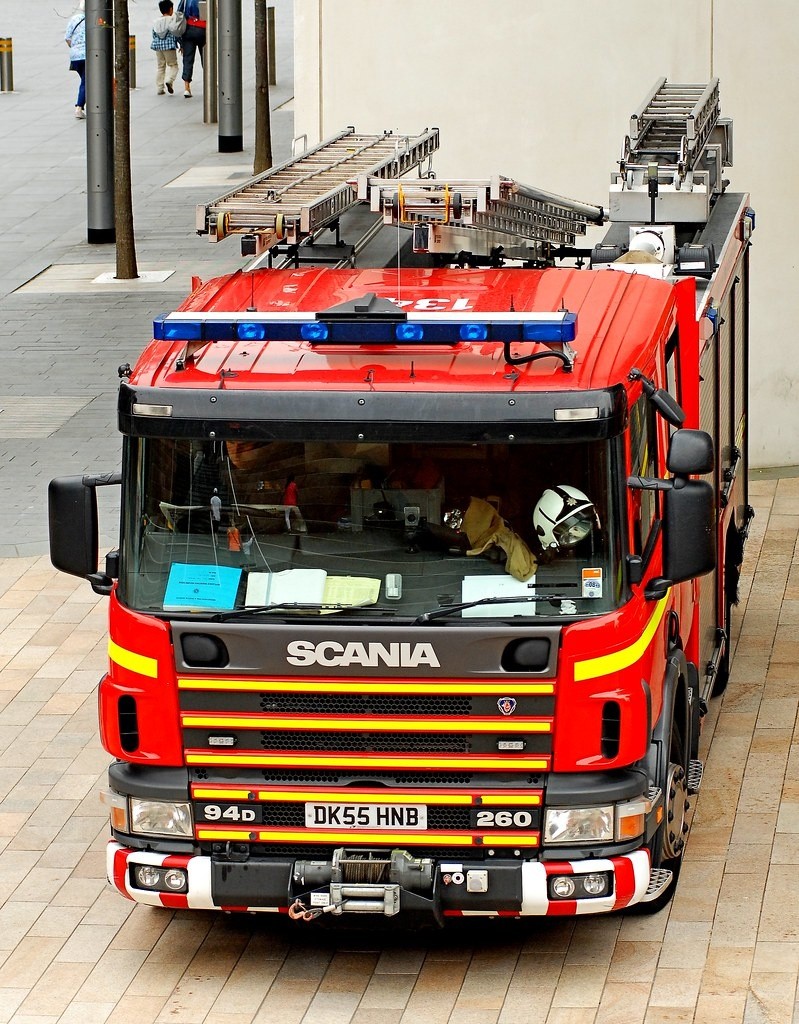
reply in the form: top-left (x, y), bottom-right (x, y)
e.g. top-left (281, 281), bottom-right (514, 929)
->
top-left (46, 73), bottom-right (757, 930)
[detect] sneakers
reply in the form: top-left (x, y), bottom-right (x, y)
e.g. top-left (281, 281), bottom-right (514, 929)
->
top-left (74, 109), bottom-right (85, 118)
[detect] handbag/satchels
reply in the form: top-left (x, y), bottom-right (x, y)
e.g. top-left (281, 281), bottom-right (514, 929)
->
top-left (168, 0), bottom-right (187, 36)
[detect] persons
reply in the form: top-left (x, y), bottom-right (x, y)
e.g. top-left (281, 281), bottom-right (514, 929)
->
top-left (151, 1), bottom-right (183, 94)
top-left (329, 443), bottom-right (540, 581)
top-left (201, 422), bottom-right (313, 533)
top-left (64, 0), bottom-right (87, 121)
top-left (176, 0), bottom-right (209, 99)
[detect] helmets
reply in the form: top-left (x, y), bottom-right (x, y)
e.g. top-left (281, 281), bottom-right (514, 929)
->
top-left (534, 485), bottom-right (601, 556)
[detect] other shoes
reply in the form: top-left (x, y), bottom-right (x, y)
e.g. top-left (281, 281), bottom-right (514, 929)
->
top-left (183, 91), bottom-right (192, 97)
top-left (166, 83), bottom-right (173, 93)
top-left (159, 90), bottom-right (164, 94)
